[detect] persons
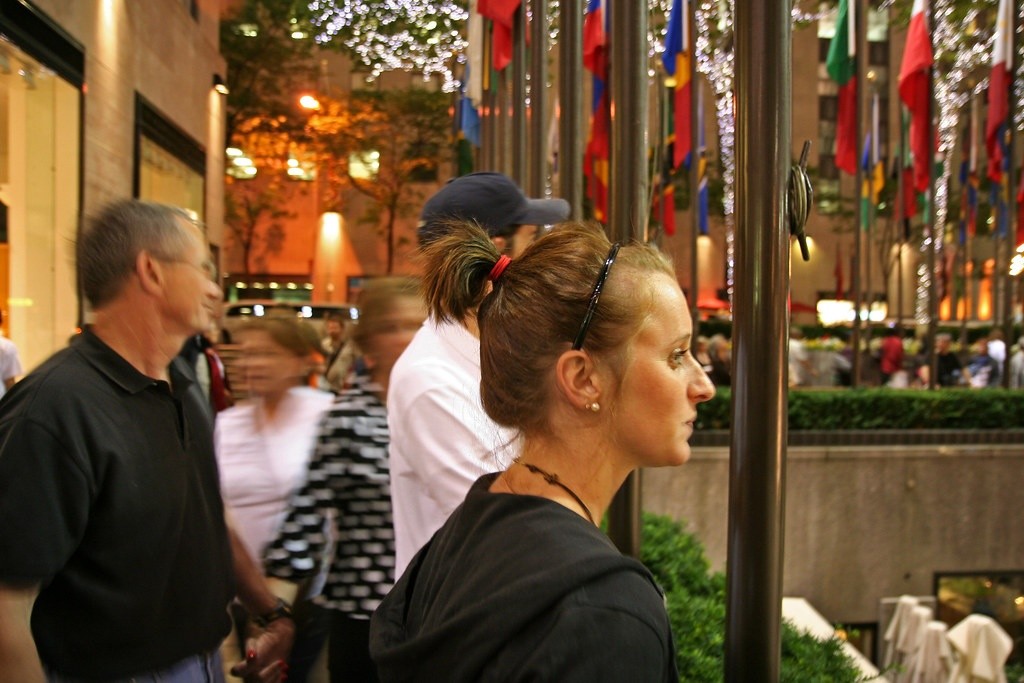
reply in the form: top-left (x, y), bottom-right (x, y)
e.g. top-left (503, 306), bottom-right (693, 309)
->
top-left (789, 322), bottom-right (1023, 390)
top-left (694, 287), bottom-right (732, 388)
top-left (1, 171), bottom-right (570, 683)
top-left (369, 221), bottom-right (716, 682)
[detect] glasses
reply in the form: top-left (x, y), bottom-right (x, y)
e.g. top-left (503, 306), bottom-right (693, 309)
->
top-left (152, 259), bottom-right (217, 279)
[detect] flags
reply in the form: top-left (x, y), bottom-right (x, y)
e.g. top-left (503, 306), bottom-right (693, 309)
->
top-left (459, 1), bottom-right (1024, 245)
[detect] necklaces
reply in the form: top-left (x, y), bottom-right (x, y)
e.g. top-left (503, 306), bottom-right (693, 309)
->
top-left (513, 457), bottom-right (596, 523)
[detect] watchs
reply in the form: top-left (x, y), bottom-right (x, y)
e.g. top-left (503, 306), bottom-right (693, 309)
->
top-left (254, 597), bottom-right (285, 627)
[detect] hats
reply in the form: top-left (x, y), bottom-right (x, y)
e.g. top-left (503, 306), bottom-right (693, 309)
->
top-left (418, 171), bottom-right (570, 238)
